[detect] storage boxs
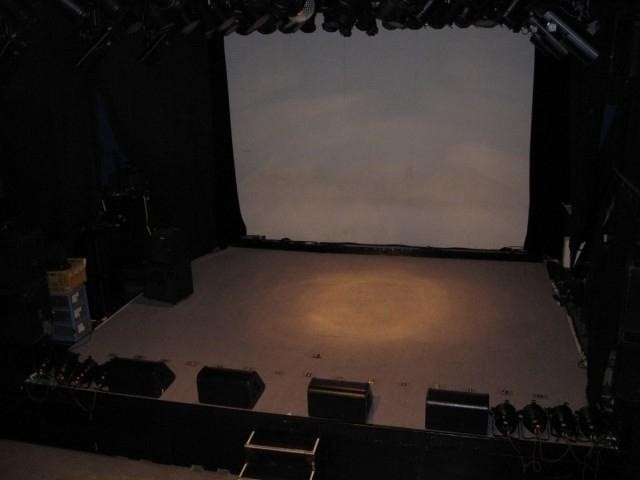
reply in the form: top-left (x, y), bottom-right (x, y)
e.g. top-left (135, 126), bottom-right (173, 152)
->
top-left (46, 256), bottom-right (92, 343)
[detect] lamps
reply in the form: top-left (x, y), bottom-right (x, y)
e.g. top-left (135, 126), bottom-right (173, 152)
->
top-left (491, 401), bottom-right (615, 443)
top-left (40, 355), bottom-right (119, 389)
